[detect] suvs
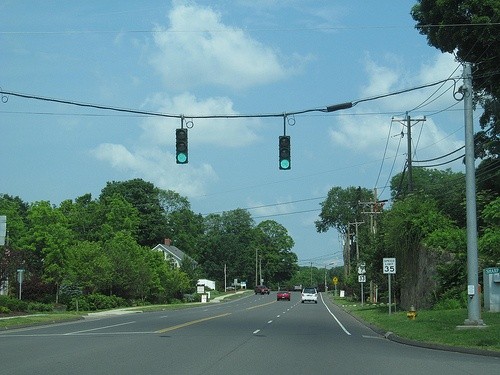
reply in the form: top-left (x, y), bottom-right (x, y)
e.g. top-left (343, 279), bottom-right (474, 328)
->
top-left (255, 285), bottom-right (269, 295)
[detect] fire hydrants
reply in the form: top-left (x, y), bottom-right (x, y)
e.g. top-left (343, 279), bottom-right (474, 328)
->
top-left (407, 304), bottom-right (418, 319)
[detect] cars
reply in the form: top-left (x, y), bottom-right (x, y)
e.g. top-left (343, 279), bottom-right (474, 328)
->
top-left (301, 287), bottom-right (318, 304)
top-left (277, 291), bottom-right (290, 301)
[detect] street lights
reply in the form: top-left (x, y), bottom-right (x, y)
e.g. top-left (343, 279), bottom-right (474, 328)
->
top-left (324, 262), bottom-right (334, 292)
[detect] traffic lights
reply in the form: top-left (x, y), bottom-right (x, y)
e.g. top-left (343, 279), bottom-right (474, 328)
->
top-left (175, 128), bottom-right (189, 164)
top-left (278, 136), bottom-right (291, 171)
